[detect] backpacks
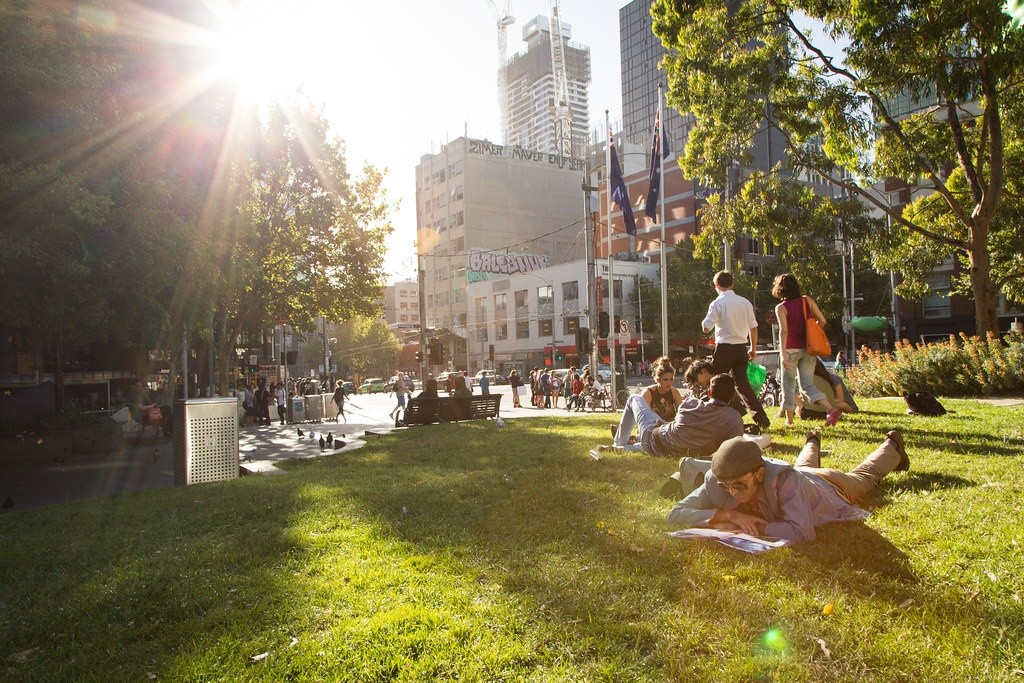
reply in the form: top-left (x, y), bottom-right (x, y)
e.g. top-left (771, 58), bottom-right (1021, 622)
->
top-left (392, 379), bottom-right (400, 391)
top-left (903, 391), bottom-right (946, 416)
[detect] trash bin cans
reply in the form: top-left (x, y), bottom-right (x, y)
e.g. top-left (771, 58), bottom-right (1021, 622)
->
top-left (172, 398), bottom-right (240, 484)
top-left (287, 395), bottom-right (306, 424)
top-left (306, 395), bottom-right (322, 422)
top-left (321, 393), bottom-right (338, 419)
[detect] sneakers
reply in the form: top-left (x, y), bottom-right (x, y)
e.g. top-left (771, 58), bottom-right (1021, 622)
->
top-left (824, 407), bottom-right (842, 427)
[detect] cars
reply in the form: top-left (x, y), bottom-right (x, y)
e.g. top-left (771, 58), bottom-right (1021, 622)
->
top-left (342, 369), bottom-right (502, 395)
top-left (546, 362), bottom-right (623, 396)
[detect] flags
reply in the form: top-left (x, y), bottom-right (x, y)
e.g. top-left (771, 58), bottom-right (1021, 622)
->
top-left (610, 126), bottom-right (636, 237)
top-left (645, 103), bottom-right (670, 224)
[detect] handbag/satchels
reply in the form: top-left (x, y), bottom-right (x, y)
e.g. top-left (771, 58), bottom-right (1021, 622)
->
top-left (335, 398), bottom-right (340, 404)
top-left (148, 407), bottom-right (163, 422)
top-left (278, 406), bottom-right (287, 416)
top-left (747, 358), bottom-right (766, 396)
top-left (805, 318), bottom-right (832, 356)
top-left (243, 402), bottom-right (248, 409)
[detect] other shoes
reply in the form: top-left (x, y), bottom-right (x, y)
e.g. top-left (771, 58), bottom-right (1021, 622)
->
top-left (574, 409), bottom-right (584, 412)
top-left (807, 430), bottom-right (821, 467)
top-left (756, 415), bottom-right (770, 429)
top-left (389, 414), bottom-right (394, 419)
top-left (595, 445), bottom-right (625, 454)
top-left (886, 430), bottom-right (909, 471)
top-left (610, 424), bottom-right (636, 445)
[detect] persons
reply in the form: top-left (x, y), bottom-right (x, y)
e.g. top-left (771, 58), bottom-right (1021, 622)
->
top-left (702, 270), bottom-right (771, 430)
top-left (529, 366), bottom-right (612, 413)
top-left (666, 429), bottom-right (910, 545)
top-left (834, 344), bottom-right (872, 373)
top-left (388, 373), bottom-right (414, 420)
top-left (244, 377), bottom-right (314, 426)
top-left (595, 356), bottom-right (744, 460)
top-left (510, 370), bottom-right (522, 407)
top-left (127, 379), bottom-right (174, 439)
top-left (776, 356), bottom-right (858, 421)
top-left (416, 380), bottom-right (437, 398)
top-left (771, 274), bottom-right (841, 427)
top-left (330, 380), bottom-right (350, 424)
top-left (445, 371), bottom-right (491, 397)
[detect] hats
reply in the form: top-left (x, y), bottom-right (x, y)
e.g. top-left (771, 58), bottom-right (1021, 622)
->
top-left (711, 435), bottom-right (762, 478)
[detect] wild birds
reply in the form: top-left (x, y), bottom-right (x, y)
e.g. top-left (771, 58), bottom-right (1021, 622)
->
top-left (151, 448), bottom-right (161, 463)
top-left (326, 431), bottom-right (334, 447)
top-left (318, 433), bottom-right (325, 453)
top-left (496, 417), bottom-right (505, 429)
top-left (296, 427), bottom-right (305, 438)
top-left (308, 427), bottom-right (316, 441)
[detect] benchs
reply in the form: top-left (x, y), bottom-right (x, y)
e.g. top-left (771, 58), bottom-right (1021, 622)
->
top-left (395, 393), bottom-right (505, 428)
top-left (125, 406), bottom-right (166, 447)
top-left (565, 391), bottom-right (613, 412)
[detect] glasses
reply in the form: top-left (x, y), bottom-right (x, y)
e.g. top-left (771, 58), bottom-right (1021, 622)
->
top-left (717, 471), bottom-right (758, 491)
